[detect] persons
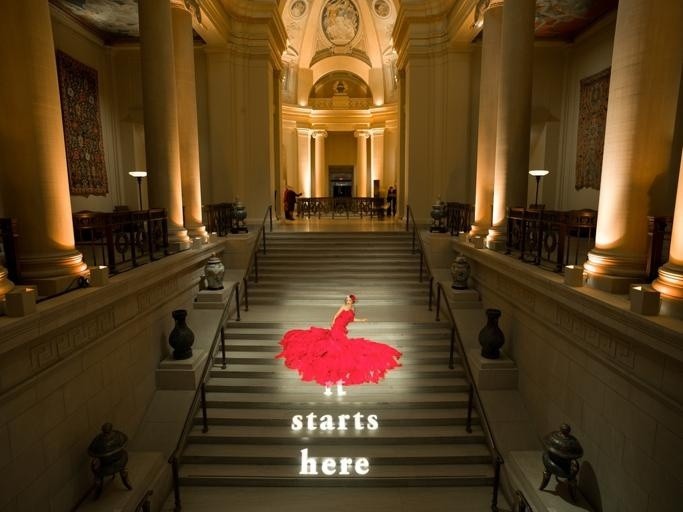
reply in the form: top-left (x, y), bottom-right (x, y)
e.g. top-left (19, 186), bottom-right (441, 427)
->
top-left (287, 189), bottom-right (303, 220)
top-left (392, 184), bottom-right (398, 216)
top-left (386, 186), bottom-right (393, 217)
top-left (283, 188), bottom-right (295, 221)
top-left (273, 294), bottom-right (404, 391)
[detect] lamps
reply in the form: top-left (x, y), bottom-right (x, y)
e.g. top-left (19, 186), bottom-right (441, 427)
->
top-left (528, 169), bottom-right (550, 209)
top-left (128, 170), bottom-right (148, 210)
